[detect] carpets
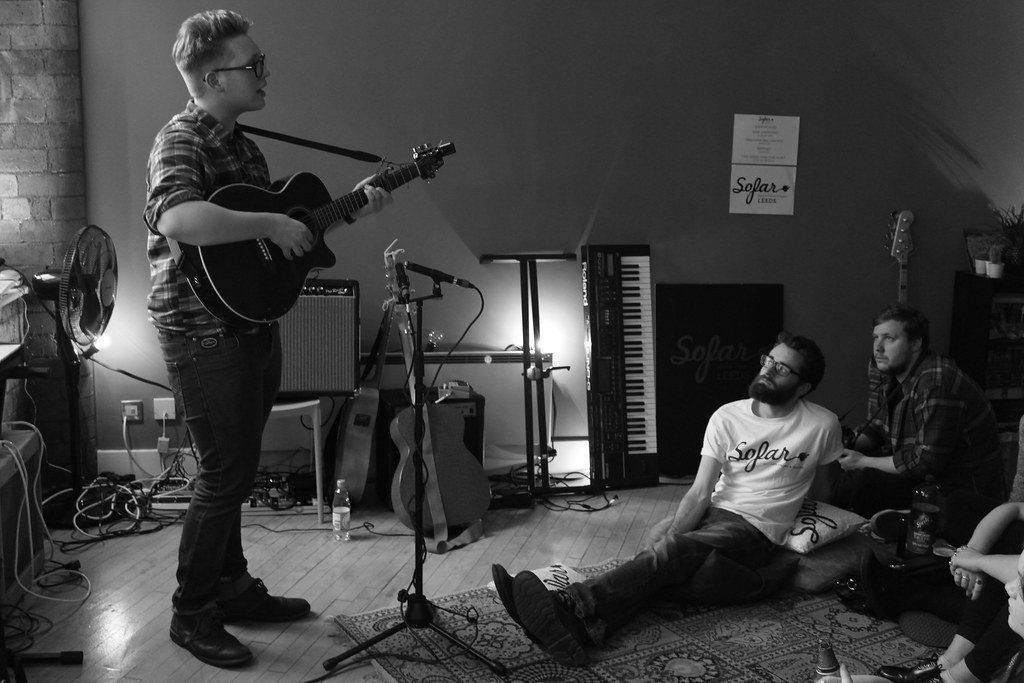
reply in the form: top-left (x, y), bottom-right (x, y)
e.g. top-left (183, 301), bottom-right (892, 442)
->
top-left (337, 553), bottom-right (951, 683)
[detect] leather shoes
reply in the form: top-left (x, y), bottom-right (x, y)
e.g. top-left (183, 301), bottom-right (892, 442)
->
top-left (169, 612), bottom-right (252, 664)
top-left (877, 653), bottom-right (943, 683)
top-left (223, 578), bottom-right (310, 622)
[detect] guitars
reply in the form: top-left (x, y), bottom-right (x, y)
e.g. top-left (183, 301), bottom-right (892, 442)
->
top-left (322, 298), bottom-right (394, 510)
top-left (186, 139), bottom-right (458, 330)
top-left (865, 208), bottom-right (917, 438)
top-left (383, 271), bottom-right (492, 538)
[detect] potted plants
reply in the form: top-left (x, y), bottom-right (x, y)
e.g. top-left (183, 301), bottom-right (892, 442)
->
top-left (966, 233), bottom-right (1012, 279)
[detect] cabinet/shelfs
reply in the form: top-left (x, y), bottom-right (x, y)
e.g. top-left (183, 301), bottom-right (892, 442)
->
top-left (952, 271), bottom-right (1024, 433)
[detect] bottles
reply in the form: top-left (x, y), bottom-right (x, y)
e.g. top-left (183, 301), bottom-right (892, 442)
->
top-left (330, 478), bottom-right (351, 542)
top-left (905, 474), bottom-right (938, 556)
top-left (816, 636), bottom-right (842, 682)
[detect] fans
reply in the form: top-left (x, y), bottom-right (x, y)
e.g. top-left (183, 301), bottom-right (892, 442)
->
top-left (31, 222), bottom-right (134, 528)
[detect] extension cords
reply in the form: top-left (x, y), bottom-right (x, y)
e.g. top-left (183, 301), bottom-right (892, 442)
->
top-left (241, 500), bottom-right (331, 515)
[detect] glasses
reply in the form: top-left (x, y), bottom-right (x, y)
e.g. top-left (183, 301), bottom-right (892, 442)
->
top-left (203, 54), bottom-right (266, 82)
top-left (760, 354), bottom-right (802, 377)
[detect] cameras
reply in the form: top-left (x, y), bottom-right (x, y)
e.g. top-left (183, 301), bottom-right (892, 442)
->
top-left (842, 425), bottom-right (857, 454)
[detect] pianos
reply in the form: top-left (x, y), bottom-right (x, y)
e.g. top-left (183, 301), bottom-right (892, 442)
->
top-left (581, 244), bottom-right (661, 493)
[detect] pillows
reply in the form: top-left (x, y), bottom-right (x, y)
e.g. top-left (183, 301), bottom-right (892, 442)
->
top-left (764, 531), bottom-right (888, 594)
top-left (778, 499), bottom-right (873, 552)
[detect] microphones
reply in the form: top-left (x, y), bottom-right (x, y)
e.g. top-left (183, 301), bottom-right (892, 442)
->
top-left (395, 262), bottom-right (409, 303)
top-left (403, 261), bottom-right (474, 289)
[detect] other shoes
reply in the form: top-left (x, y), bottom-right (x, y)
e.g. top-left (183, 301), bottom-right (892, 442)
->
top-left (492, 563), bottom-right (568, 646)
top-left (871, 509), bottom-right (911, 540)
top-left (899, 611), bottom-right (959, 648)
top-left (512, 569), bottom-right (591, 667)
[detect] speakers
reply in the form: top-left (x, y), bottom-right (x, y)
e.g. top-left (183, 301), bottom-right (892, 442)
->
top-left (375, 381), bottom-right (486, 511)
top-left (0, 429), bottom-right (46, 623)
top-left (276, 278), bottom-right (361, 400)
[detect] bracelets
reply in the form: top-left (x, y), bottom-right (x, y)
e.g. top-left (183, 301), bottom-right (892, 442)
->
top-left (343, 215), bottom-right (357, 224)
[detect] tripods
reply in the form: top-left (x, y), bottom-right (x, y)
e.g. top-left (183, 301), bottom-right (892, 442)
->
top-left (321, 284), bottom-right (508, 675)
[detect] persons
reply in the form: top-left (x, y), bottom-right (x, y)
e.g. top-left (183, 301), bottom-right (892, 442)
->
top-left (836, 301), bottom-right (1024, 683)
top-left (491, 329), bottom-right (845, 667)
top-left (140, 8), bottom-right (394, 670)
